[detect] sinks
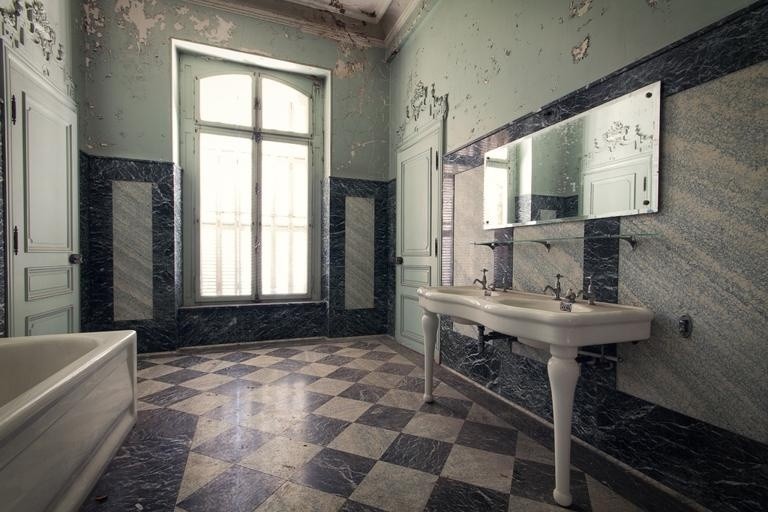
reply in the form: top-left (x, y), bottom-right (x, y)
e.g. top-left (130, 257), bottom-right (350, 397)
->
top-left (437, 287), bottom-right (493, 300)
top-left (497, 296), bottom-right (590, 314)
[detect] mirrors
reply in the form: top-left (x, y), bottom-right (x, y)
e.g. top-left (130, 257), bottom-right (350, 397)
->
top-left (483, 80), bottom-right (662, 231)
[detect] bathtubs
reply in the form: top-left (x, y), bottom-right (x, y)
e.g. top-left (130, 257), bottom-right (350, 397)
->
top-left (0, 330), bottom-right (136, 512)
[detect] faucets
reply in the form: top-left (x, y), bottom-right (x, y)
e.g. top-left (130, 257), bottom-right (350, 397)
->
top-left (473, 268), bottom-right (490, 291)
top-left (575, 274), bottom-right (596, 303)
top-left (502, 270), bottom-right (511, 293)
top-left (543, 273), bottom-right (564, 301)
